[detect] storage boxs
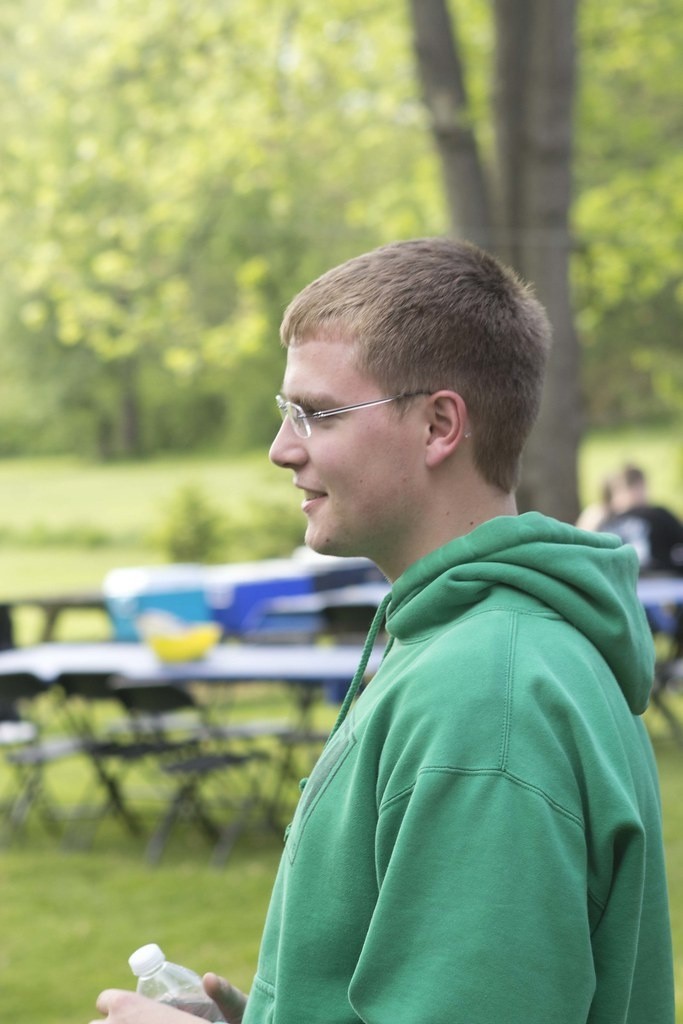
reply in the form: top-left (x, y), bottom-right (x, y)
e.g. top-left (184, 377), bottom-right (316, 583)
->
top-left (107, 560), bottom-right (320, 642)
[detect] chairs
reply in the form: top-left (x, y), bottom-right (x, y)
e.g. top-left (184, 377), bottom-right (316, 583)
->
top-left (0, 597), bottom-right (328, 872)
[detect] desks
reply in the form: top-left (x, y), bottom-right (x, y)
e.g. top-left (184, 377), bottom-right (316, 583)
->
top-left (300, 582), bottom-right (683, 738)
top-left (0, 641), bottom-right (385, 872)
top-left (5, 592), bottom-right (109, 641)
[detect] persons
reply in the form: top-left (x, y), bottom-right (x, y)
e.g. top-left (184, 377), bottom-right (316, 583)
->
top-left (84, 238), bottom-right (677, 1024)
top-left (575, 463), bottom-right (683, 662)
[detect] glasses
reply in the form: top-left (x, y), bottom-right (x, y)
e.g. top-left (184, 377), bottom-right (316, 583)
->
top-left (275, 390), bottom-right (432, 440)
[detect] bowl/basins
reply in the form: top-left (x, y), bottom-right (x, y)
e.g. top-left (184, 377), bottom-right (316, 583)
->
top-left (147, 622), bottom-right (221, 663)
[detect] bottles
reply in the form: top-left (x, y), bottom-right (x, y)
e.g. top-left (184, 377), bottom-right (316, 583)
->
top-left (128, 943), bottom-right (230, 1024)
